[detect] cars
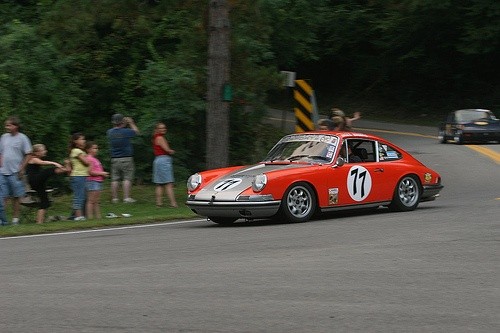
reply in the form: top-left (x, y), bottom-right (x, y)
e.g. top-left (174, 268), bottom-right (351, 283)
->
top-left (438, 108), bottom-right (499, 145)
top-left (184, 130), bottom-right (444, 226)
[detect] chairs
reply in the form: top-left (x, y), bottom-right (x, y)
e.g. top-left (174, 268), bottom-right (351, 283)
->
top-left (19, 175), bottom-right (59, 223)
top-left (353, 148), bottom-right (369, 162)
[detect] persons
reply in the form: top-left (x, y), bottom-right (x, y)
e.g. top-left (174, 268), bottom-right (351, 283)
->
top-left (68, 133), bottom-right (90, 221)
top-left (152, 123), bottom-right (178, 208)
top-left (26, 144), bottom-right (71, 224)
top-left (0, 116), bottom-right (33, 224)
top-left (339, 140), bottom-right (361, 163)
top-left (318, 109), bottom-right (360, 132)
top-left (85, 141), bottom-right (109, 219)
top-left (106, 114), bottom-right (138, 203)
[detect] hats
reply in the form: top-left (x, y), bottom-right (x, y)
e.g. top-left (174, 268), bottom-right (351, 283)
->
top-left (111, 113), bottom-right (125, 125)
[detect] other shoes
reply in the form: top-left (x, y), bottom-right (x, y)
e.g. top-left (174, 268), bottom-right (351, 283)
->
top-left (73, 216), bottom-right (86, 221)
top-left (0, 219), bottom-right (8, 225)
top-left (124, 198), bottom-right (137, 203)
top-left (11, 222), bottom-right (20, 225)
top-left (112, 198), bottom-right (120, 203)
